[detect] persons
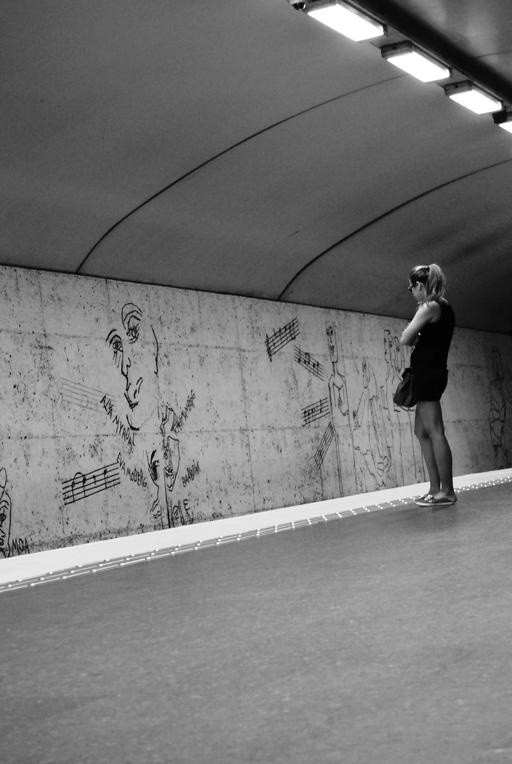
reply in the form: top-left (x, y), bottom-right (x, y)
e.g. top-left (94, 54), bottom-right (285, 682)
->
top-left (399, 262), bottom-right (459, 507)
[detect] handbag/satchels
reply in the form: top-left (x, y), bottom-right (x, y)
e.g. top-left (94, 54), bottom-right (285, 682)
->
top-left (393, 368), bottom-right (418, 407)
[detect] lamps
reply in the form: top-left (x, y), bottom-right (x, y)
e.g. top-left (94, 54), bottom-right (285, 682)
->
top-left (297, 0), bottom-right (512, 134)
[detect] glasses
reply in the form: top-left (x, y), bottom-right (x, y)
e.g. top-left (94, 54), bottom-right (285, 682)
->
top-left (408, 284), bottom-right (415, 292)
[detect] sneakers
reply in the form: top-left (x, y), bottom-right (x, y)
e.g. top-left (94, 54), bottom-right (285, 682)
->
top-left (414, 494), bottom-right (455, 507)
